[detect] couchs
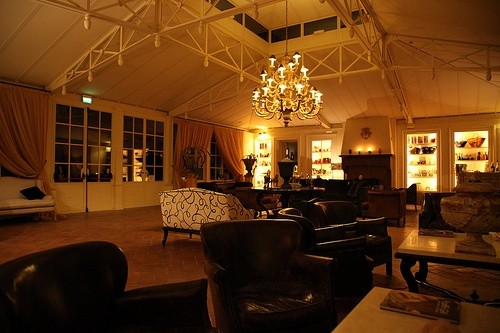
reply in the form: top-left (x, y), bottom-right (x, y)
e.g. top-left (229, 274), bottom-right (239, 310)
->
top-left (0, 179), bottom-right (500, 333)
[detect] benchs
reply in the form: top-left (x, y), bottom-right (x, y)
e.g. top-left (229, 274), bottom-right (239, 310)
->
top-left (0, 177), bottom-right (56, 221)
top-left (158, 186), bottom-right (255, 248)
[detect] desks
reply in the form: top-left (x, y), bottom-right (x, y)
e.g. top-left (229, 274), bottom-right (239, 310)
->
top-left (332, 286), bottom-right (500, 333)
top-left (394, 230), bottom-right (500, 309)
top-left (249, 186), bottom-right (325, 208)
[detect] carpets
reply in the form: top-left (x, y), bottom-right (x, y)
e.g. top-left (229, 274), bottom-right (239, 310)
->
top-left (372, 259), bottom-right (500, 303)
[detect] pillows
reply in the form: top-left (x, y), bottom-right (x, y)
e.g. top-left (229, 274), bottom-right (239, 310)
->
top-left (20, 186), bottom-right (46, 200)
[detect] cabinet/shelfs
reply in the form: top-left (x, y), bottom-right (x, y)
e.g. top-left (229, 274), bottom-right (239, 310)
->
top-left (339, 154), bottom-right (395, 203)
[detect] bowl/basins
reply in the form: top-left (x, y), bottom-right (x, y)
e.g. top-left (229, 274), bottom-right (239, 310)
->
top-left (456, 140), bottom-right (467, 147)
top-left (422, 146), bottom-right (435, 154)
top-left (467, 138), bottom-right (485, 148)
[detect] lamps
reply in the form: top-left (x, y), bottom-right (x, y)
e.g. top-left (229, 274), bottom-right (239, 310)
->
top-left (251, 0), bottom-right (323, 127)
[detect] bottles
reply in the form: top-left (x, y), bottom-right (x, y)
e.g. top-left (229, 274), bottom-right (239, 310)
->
top-left (476, 151), bottom-right (488, 160)
top-left (485, 162), bottom-right (490, 172)
top-left (490, 162), bottom-right (495, 172)
top-left (495, 160), bottom-right (500, 173)
top-left (455, 153), bottom-right (474, 160)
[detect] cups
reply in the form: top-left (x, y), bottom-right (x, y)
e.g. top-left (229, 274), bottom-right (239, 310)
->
top-left (412, 136), bottom-right (428, 144)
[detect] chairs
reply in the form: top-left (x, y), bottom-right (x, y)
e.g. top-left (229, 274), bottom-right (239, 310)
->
top-left (360, 188), bottom-right (406, 228)
top-left (400, 184), bottom-right (417, 212)
